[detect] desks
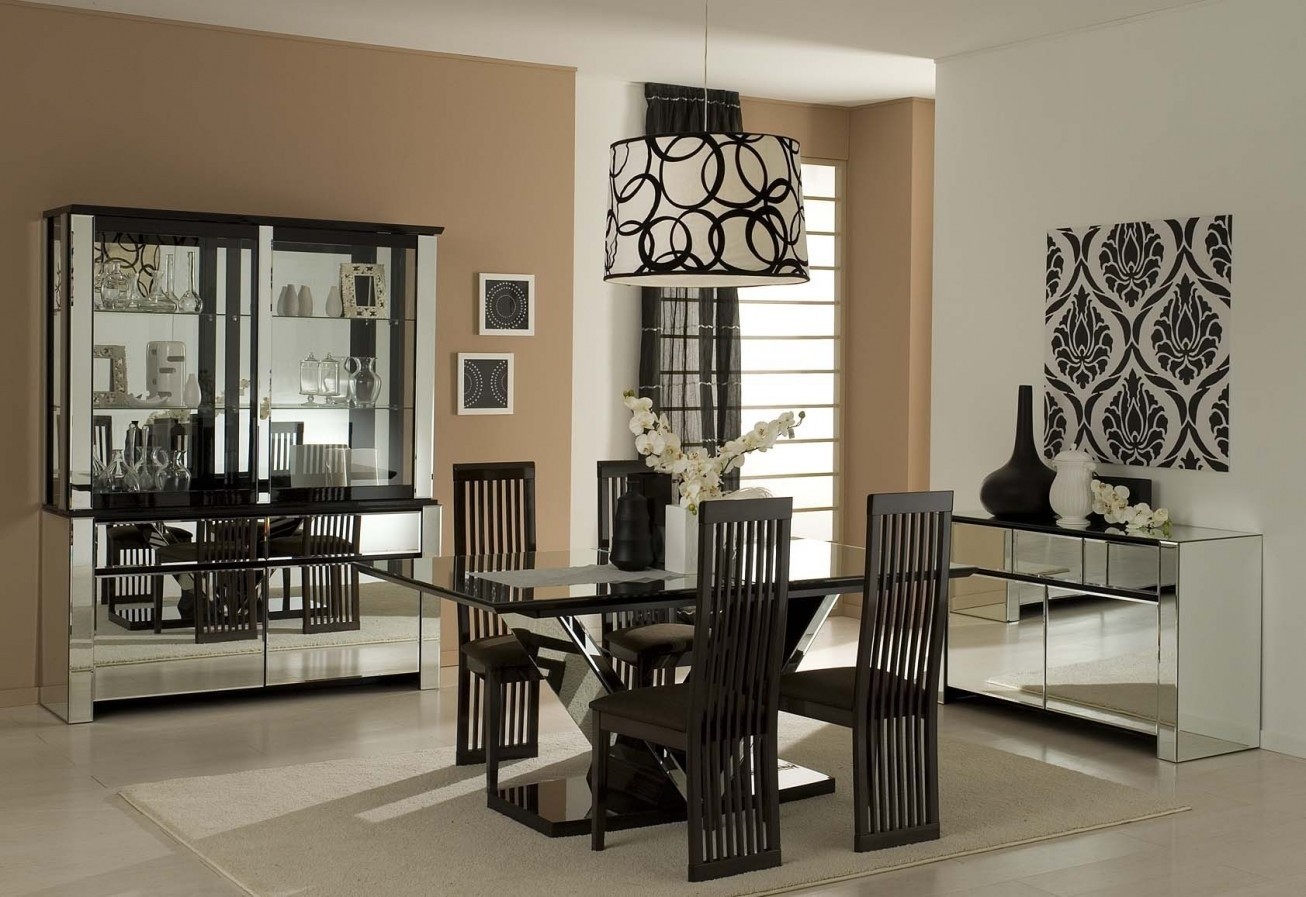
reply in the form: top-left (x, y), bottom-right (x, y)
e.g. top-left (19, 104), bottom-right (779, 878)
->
top-left (351, 537), bottom-right (977, 841)
top-left (72, 463), bottom-right (396, 633)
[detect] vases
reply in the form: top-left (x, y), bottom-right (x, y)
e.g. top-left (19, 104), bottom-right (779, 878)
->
top-left (183, 373), bottom-right (202, 408)
top-left (978, 385), bottom-right (1056, 521)
top-left (664, 503), bottom-right (715, 574)
top-left (276, 283), bottom-right (342, 317)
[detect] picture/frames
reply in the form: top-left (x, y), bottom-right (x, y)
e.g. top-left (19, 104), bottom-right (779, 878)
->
top-left (338, 262), bottom-right (389, 320)
top-left (455, 351), bottom-right (515, 416)
top-left (478, 270), bottom-right (535, 337)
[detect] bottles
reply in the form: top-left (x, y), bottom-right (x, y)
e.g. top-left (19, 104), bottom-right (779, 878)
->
top-left (95, 251), bottom-right (204, 314)
top-left (349, 356), bottom-right (382, 407)
top-left (155, 450), bottom-right (191, 493)
top-left (1048, 443), bottom-right (1097, 529)
top-left (183, 374), bottom-right (202, 408)
top-left (277, 284), bottom-right (313, 317)
top-left (124, 421), bottom-right (143, 466)
top-left (298, 352), bottom-right (341, 407)
top-left (981, 384), bottom-right (1057, 524)
top-left (325, 285), bottom-right (343, 317)
top-left (609, 481), bottom-right (653, 571)
top-left (95, 449), bottom-right (140, 493)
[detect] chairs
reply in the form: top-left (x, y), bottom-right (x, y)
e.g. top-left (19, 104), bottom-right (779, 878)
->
top-left (778, 488), bottom-right (955, 854)
top-left (597, 459), bottom-right (677, 690)
top-left (54, 412), bottom-right (360, 645)
top-left (449, 460), bottom-right (545, 793)
top-left (589, 495), bottom-right (794, 882)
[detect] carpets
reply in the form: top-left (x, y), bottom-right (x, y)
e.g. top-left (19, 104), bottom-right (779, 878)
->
top-left (117, 710), bottom-right (1191, 897)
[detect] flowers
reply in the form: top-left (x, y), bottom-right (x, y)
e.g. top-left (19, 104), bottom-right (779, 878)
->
top-left (622, 387), bottom-right (806, 517)
top-left (1091, 479), bottom-right (1172, 540)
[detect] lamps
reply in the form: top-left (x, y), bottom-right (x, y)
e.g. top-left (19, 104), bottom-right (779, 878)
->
top-left (604, 0), bottom-right (812, 287)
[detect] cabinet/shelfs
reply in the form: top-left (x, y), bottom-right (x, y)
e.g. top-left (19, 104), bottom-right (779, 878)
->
top-left (41, 201), bottom-right (448, 715)
top-left (938, 511), bottom-right (1264, 764)
